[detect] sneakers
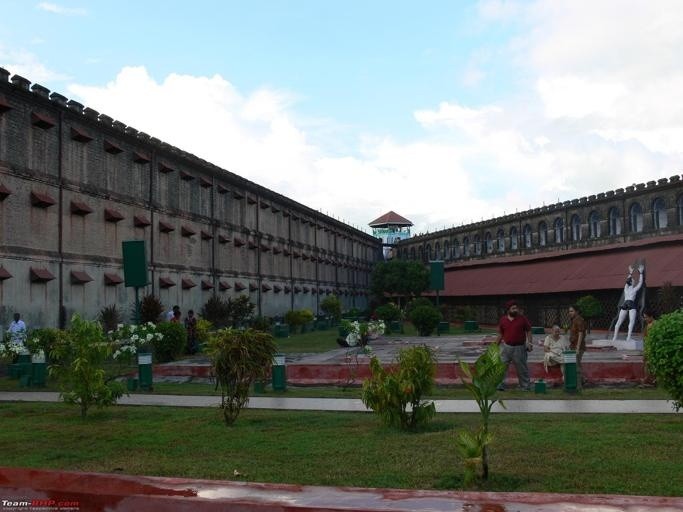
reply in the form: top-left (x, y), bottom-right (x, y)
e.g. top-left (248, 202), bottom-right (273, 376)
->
top-left (522, 386), bottom-right (531, 391)
top-left (496, 385), bottom-right (504, 390)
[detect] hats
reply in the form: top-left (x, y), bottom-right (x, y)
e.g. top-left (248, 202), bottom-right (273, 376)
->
top-left (504, 300), bottom-right (516, 309)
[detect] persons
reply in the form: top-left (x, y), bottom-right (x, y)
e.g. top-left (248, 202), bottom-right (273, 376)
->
top-left (7, 313), bottom-right (26, 333)
top-left (494, 300), bottom-right (532, 391)
top-left (568, 306), bottom-right (586, 388)
top-left (167, 305), bottom-right (198, 325)
top-left (543, 324), bottom-right (566, 383)
top-left (612, 264), bottom-right (645, 342)
top-left (640, 308), bottom-right (660, 385)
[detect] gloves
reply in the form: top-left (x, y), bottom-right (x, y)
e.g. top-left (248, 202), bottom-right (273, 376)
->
top-left (527, 343), bottom-right (532, 352)
